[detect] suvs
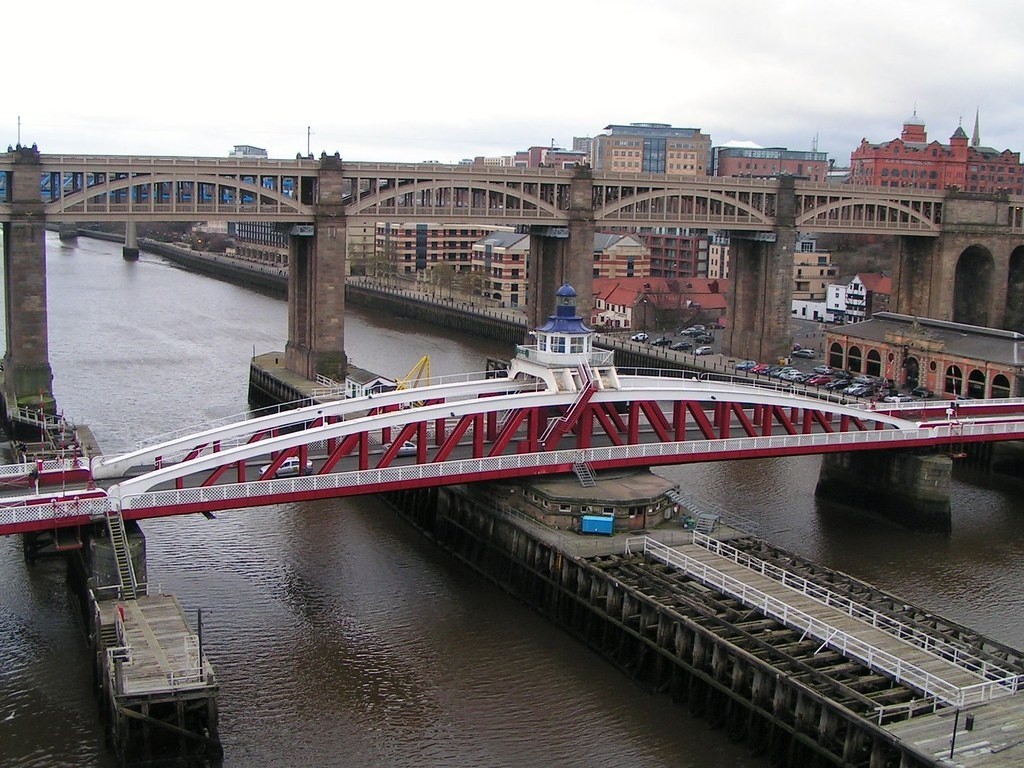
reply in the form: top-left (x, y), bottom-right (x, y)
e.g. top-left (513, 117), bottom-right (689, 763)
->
top-left (792, 349), bottom-right (816, 359)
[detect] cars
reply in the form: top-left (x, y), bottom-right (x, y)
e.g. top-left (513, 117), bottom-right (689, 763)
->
top-left (693, 346), bottom-right (712, 356)
top-left (669, 341), bottom-right (692, 351)
top-left (650, 337), bottom-right (672, 347)
top-left (736, 360), bottom-right (898, 402)
top-left (259, 456), bottom-right (314, 479)
top-left (884, 393), bottom-right (913, 403)
top-left (909, 387), bottom-right (933, 398)
top-left (795, 344), bottom-right (802, 350)
top-left (382, 440), bottom-right (418, 459)
top-left (681, 324), bottom-right (712, 345)
top-left (631, 332), bottom-right (649, 342)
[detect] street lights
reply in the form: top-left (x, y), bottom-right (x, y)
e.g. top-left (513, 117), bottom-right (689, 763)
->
top-left (643, 298), bottom-right (648, 344)
